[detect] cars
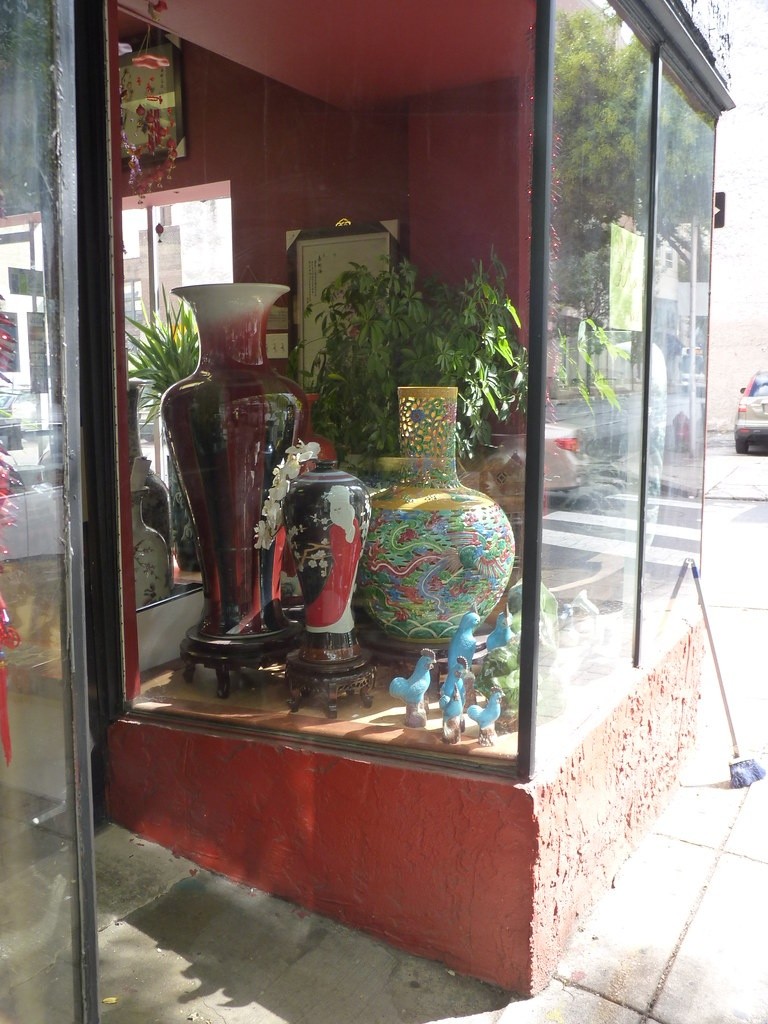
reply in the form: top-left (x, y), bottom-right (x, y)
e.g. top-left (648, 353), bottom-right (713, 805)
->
top-left (1, 384), bottom-right (166, 441)
top-left (542, 416), bottom-right (608, 492)
top-left (735, 369), bottom-right (768, 453)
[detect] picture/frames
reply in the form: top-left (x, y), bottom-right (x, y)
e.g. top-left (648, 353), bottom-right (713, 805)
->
top-left (284, 219), bottom-right (401, 395)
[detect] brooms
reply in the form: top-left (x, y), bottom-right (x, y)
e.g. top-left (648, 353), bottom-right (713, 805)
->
top-left (689, 557), bottom-right (765, 789)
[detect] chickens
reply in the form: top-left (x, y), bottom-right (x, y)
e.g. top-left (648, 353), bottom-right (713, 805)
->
top-left (388, 602), bottom-right (517, 730)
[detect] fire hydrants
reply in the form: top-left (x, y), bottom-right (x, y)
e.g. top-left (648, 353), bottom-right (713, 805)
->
top-left (673, 412), bottom-right (691, 453)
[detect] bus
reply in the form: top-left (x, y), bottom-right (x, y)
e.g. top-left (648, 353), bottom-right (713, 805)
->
top-left (679, 346), bottom-right (706, 394)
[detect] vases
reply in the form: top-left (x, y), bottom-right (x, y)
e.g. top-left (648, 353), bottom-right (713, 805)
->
top-left (351, 387), bottom-right (516, 647)
top-left (130, 486), bottom-right (174, 609)
top-left (281, 460), bottom-right (373, 664)
top-left (159, 282), bottom-right (308, 640)
